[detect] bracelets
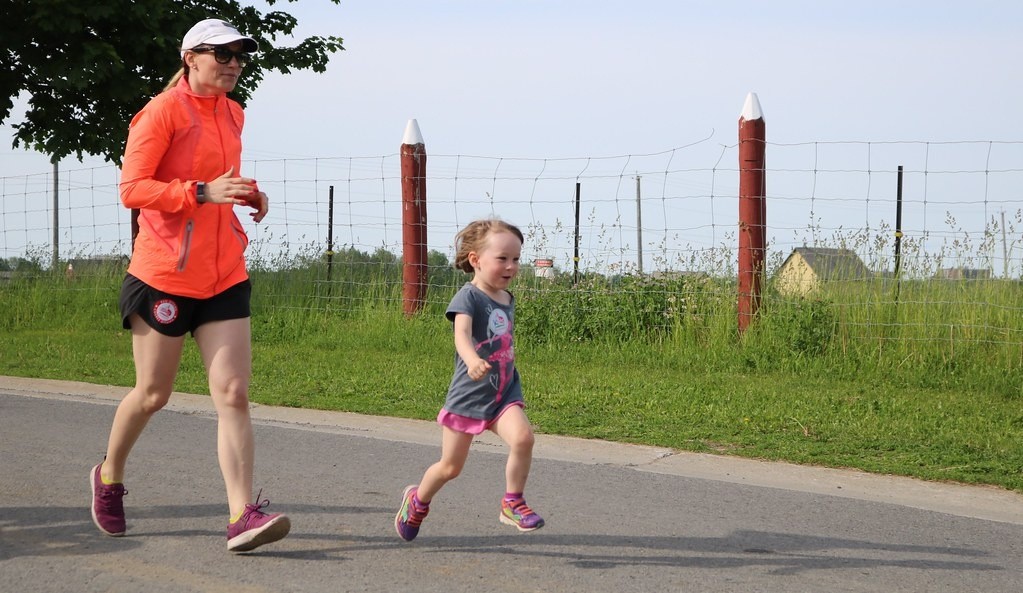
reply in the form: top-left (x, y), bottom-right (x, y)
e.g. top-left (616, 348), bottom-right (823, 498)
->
top-left (196, 181), bottom-right (206, 203)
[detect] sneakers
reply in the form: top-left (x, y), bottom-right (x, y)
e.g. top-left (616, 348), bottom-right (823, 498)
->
top-left (226, 488), bottom-right (291, 551)
top-left (394, 485), bottom-right (429, 542)
top-left (499, 498), bottom-right (545, 532)
top-left (89, 456), bottom-right (129, 537)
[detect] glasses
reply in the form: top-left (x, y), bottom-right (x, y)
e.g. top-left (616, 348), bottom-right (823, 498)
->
top-left (192, 45), bottom-right (253, 68)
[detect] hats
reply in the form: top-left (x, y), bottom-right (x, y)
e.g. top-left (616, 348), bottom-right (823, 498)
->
top-left (180, 18), bottom-right (259, 61)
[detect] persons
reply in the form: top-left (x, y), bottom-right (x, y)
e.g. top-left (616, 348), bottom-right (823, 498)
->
top-left (395, 220), bottom-right (545, 541)
top-left (91, 21), bottom-right (291, 552)
